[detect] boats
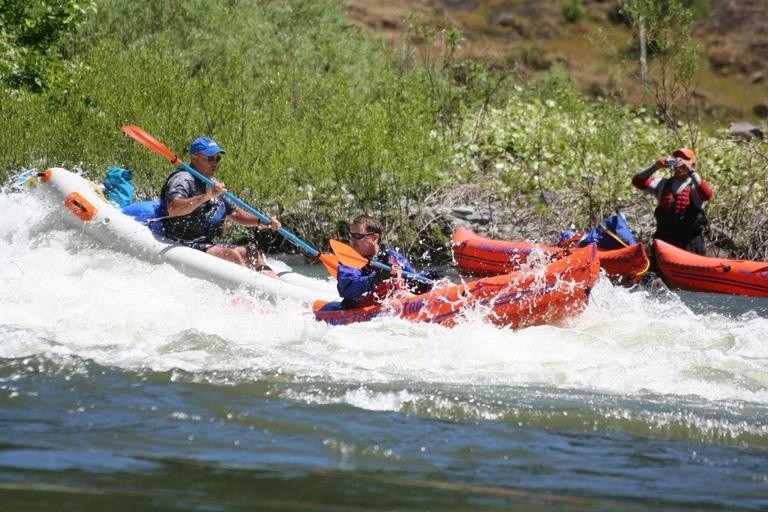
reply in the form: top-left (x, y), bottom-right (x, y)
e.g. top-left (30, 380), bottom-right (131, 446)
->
top-left (647, 234), bottom-right (767, 299)
top-left (445, 220), bottom-right (652, 292)
top-left (28, 158), bottom-right (325, 311)
top-left (311, 241), bottom-right (610, 334)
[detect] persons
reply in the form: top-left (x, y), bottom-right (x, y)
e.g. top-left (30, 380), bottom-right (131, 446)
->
top-left (159, 135), bottom-right (283, 279)
top-left (336, 216), bottom-right (440, 310)
top-left (631, 147), bottom-right (717, 255)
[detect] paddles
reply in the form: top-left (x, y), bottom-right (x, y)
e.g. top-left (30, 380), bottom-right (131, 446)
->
top-left (121, 124), bottom-right (340, 279)
top-left (329, 238), bottom-right (435, 288)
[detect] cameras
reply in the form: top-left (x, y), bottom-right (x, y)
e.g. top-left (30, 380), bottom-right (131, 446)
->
top-left (665, 159), bottom-right (677, 165)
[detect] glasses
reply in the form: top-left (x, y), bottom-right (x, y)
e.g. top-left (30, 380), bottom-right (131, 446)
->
top-left (349, 230), bottom-right (374, 241)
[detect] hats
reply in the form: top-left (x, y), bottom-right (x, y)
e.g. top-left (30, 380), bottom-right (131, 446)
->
top-left (672, 147), bottom-right (696, 160)
top-left (187, 135), bottom-right (226, 157)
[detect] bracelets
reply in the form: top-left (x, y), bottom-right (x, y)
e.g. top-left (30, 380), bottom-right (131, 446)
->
top-left (687, 170), bottom-right (695, 176)
top-left (257, 218), bottom-right (262, 227)
